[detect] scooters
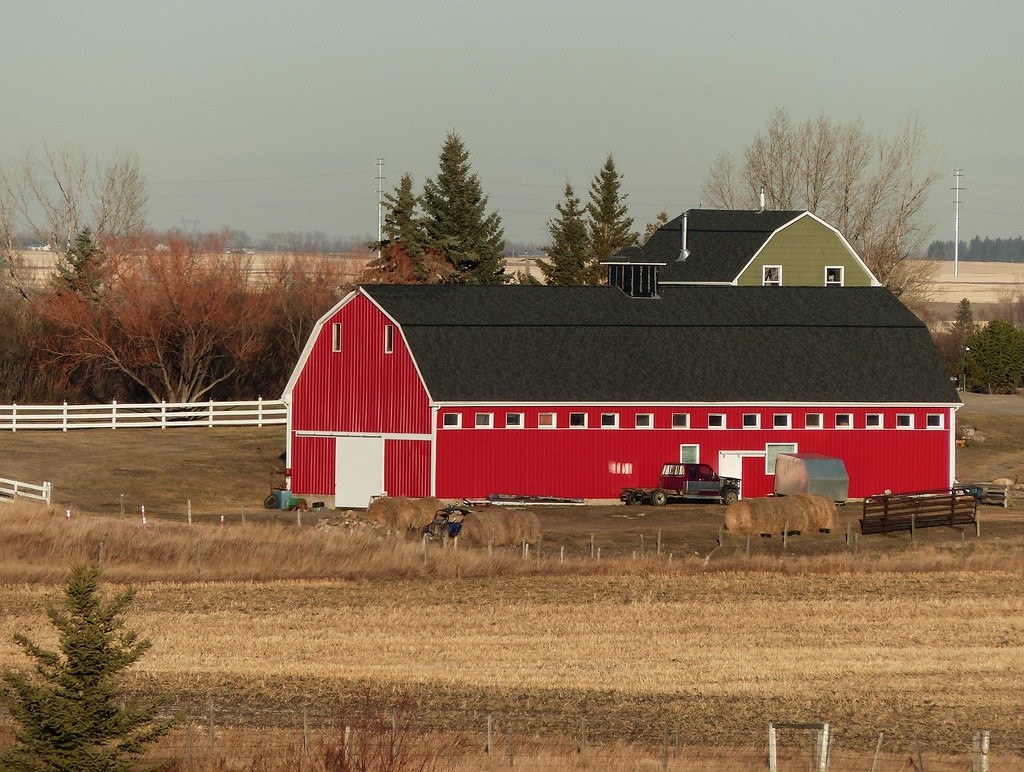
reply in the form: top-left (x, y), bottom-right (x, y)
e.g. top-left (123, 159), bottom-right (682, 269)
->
top-left (426, 508), bottom-right (473, 549)
